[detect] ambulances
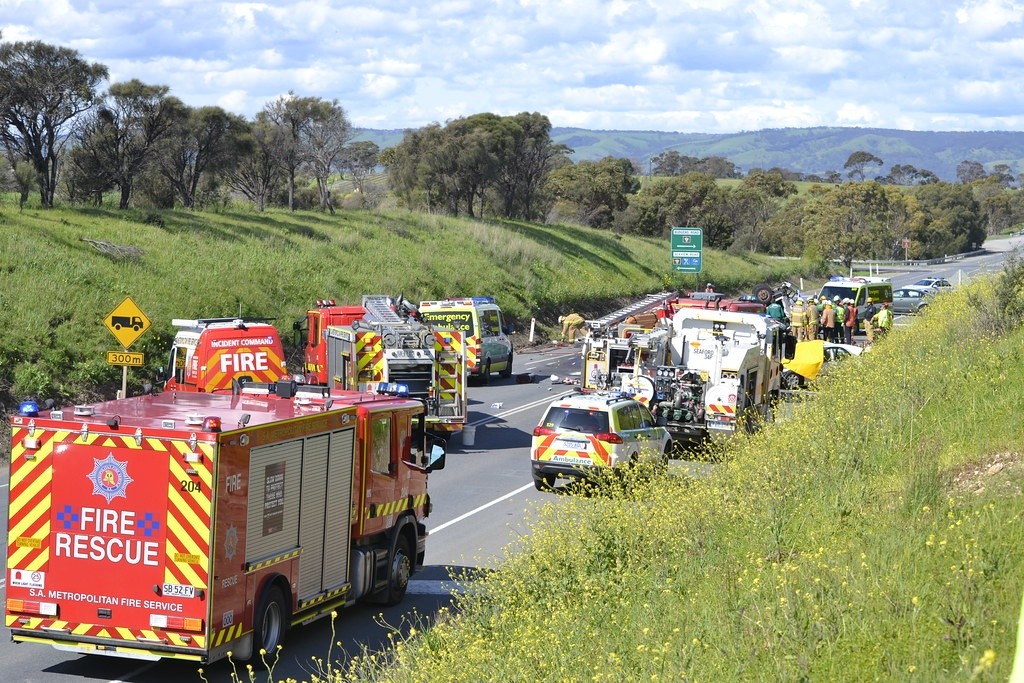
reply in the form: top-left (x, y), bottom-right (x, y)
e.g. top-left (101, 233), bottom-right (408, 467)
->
top-left (816, 277), bottom-right (893, 334)
top-left (418, 297), bottom-right (513, 381)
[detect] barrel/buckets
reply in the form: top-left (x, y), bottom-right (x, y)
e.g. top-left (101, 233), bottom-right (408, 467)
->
top-left (462, 426), bottom-right (476, 445)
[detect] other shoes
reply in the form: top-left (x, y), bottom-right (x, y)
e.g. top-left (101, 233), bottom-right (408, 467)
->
top-left (568, 341), bottom-right (574, 344)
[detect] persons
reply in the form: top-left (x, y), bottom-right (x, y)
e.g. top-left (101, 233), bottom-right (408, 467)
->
top-left (557, 313), bottom-right (591, 343)
top-left (790, 295), bottom-right (859, 345)
top-left (863, 297), bottom-right (893, 345)
top-left (705, 282), bottom-right (714, 293)
top-left (765, 298), bottom-right (785, 322)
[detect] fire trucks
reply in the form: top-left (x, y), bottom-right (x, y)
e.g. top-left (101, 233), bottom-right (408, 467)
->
top-left (6, 380), bottom-right (446, 670)
top-left (163, 318), bottom-right (289, 397)
top-left (293, 291), bottom-right (466, 432)
top-left (582, 291), bottom-right (785, 452)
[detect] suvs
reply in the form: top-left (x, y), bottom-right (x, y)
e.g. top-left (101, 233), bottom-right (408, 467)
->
top-left (531, 388), bottom-right (673, 498)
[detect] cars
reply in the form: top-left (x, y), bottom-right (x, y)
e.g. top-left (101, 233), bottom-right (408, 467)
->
top-left (915, 279), bottom-right (952, 294)
top-left (892, 286), bottom-right (941, 312)
top-left (780, 342), bottom-right (863, 389)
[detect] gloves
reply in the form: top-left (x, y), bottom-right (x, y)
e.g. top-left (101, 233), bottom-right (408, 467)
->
top-left (841, 323), bottom-right (844, 327)
top-left (870, 317), bottom-right (875, 323)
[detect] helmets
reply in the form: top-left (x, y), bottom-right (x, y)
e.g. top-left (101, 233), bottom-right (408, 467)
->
top-left (558, 316), bottom-right (562, 323)
top-left (850, 299), bottom-right (855, 304)
top-left (820, 296), bottom-right (826, 301)
top-left (867, 297), bottom-right (873, 302)
top-left (834, 295), bottom-right (840, 301)
top-left (843, 297), bottom-right (850, 303)
top-left (880, 303), bottom-right (885, 309)
top-left (807, 297), bottom-right (814, 301)
top-left (826, 300), bottom-right (832, 306)
top-left (796, 299), bottom-right (803, 306)
